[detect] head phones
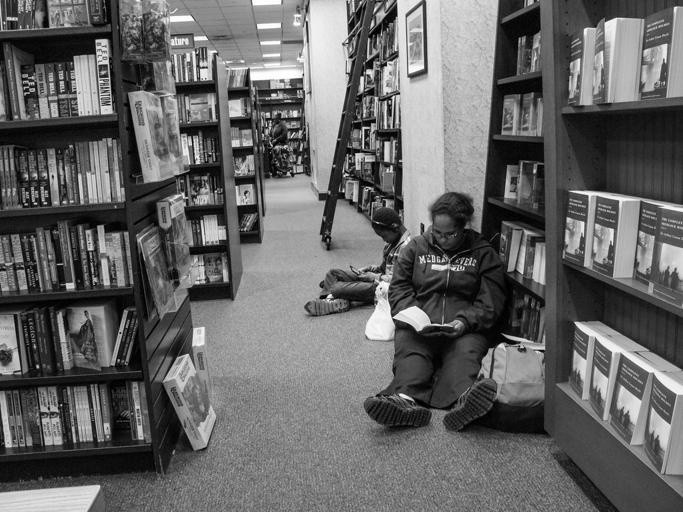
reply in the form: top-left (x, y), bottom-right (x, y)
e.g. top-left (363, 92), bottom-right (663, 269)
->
top-left (371, 219), bottom-right (400, 233)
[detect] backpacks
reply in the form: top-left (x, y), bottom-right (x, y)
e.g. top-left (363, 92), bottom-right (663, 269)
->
top-left (475, 342), bottom-right (545, 428)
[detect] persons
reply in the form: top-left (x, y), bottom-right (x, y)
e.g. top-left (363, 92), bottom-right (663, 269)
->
top-left (298, 207), bottom-right (410, 319)
top-left (361, 192), bottom-right (506, 434)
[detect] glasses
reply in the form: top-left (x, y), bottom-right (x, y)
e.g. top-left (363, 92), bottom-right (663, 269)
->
top-left (432, 229), bottom-right (458, 239)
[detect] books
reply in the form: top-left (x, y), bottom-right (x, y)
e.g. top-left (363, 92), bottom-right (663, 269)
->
top-left (564, 2), bottom-right (681, 101)
top-left (560, 187), bottom-right (681, 309)
top-left (1, 2), bottom-right (155, 453)
top-left (129, 45), bottom-right (232, 454)
top-left (341, 0), bottom-right (404, 229)
top-left (502, 252), bottom-right (546, 354)
top-left (394, 304), bottom-right (466, 339)
top-left (559, 314), bottom-right (681, 478)
top-left (490, 26), bottom-right (547, 249)
top-left (219, 62), bottom-right (308, 241)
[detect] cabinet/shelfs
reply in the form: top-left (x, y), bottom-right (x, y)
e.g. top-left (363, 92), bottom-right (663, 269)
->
top-left (340, 0), bottom-right (403, 238)
top-left (253, 78), bottom-right (308, 177)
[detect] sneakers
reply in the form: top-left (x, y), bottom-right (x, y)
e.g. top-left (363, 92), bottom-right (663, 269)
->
top-left (304, 295), bottom-right (349, 316)
top-left (363, 394), bottom-right (431, 428)
top-left (443, 379), bottom-right (497, 431)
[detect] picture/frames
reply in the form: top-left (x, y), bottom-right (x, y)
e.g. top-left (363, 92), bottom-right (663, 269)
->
top-left (405, 0), bottom-right (428, 79)
